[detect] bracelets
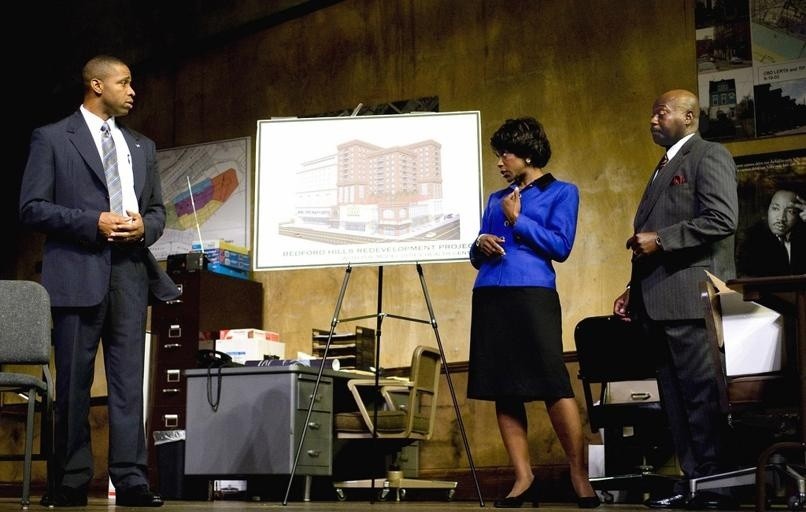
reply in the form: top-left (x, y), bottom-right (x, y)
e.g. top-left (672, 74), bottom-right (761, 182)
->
top-left (626, 285), bottom-right (631, 289)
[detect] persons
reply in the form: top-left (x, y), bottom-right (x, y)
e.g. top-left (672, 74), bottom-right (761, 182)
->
top-left (17, 55), bottom-right (181, 508)
top-left (612, 90), bottom-right (740, 510)
top-left (738, 189), bottom-right (806, 279)
top-left (466, 116), bottom-right (602, 509)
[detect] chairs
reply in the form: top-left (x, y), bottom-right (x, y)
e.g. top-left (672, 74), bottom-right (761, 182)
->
top-left (0, 279), bottom-right (56, 511)
top-left (329, 346), bottom-right (461, 500)
top-left (687, 279), bottom-right (805, 512)
top-left (572, 311), bottom-right (694, 509)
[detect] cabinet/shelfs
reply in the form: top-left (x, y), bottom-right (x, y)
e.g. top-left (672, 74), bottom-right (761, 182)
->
top-left (309, 325), bottom-right (375, 372)
top-left (155, 267), bottom-right (264, 501)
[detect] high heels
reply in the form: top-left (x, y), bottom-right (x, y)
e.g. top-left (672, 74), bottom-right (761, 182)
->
top-left (493, 476), bottom-right (539, 509)
top-left (569, 475), bottom-right (601, 509)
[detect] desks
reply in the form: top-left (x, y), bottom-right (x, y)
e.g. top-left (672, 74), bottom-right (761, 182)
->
top-left (0, 324), bottom-right (152, 501)
top-left (181, 367), bottom-right (420, 501)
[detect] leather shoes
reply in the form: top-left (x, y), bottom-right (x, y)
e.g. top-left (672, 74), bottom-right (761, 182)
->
top-left (115, 485), bottom-right (164, 507)
top-left (40, 491), bottom-right (88, 508)
top-left (642, 491), bottom-right (740, 512)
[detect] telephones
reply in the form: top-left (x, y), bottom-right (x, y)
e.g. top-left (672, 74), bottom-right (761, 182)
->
top-left (195, 349), bottom-right (232, 368)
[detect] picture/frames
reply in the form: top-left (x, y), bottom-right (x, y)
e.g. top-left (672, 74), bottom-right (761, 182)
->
top-left (727, 145), bottom-right (806, 297)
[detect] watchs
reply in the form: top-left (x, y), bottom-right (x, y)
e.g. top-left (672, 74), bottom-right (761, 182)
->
top-left (476, 233), bottom-right (485, 247)
top-left (656, 230), bottom-right (663, 247)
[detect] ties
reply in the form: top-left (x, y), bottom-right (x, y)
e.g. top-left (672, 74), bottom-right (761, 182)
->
top-left (647, 153), bottom-right (669, 192)
top-left (100, 121), bottom-right (123, 217)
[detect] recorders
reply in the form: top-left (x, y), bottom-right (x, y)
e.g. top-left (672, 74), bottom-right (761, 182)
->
top-left (166, 254), bottom-right (210, 272)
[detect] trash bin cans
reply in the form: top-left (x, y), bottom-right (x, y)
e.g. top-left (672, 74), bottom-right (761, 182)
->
top-left (153, 430), bottom-right (209, 501)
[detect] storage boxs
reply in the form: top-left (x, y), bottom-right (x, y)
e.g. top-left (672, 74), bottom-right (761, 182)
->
top-left (212, 337), bottom-right (286, 367)
top-left (189, 238), bottom-right (251, 272)
top-left (721, 290), bottom-right (786, 379)
top-left (601, 377), bottom-right (661, 435)
